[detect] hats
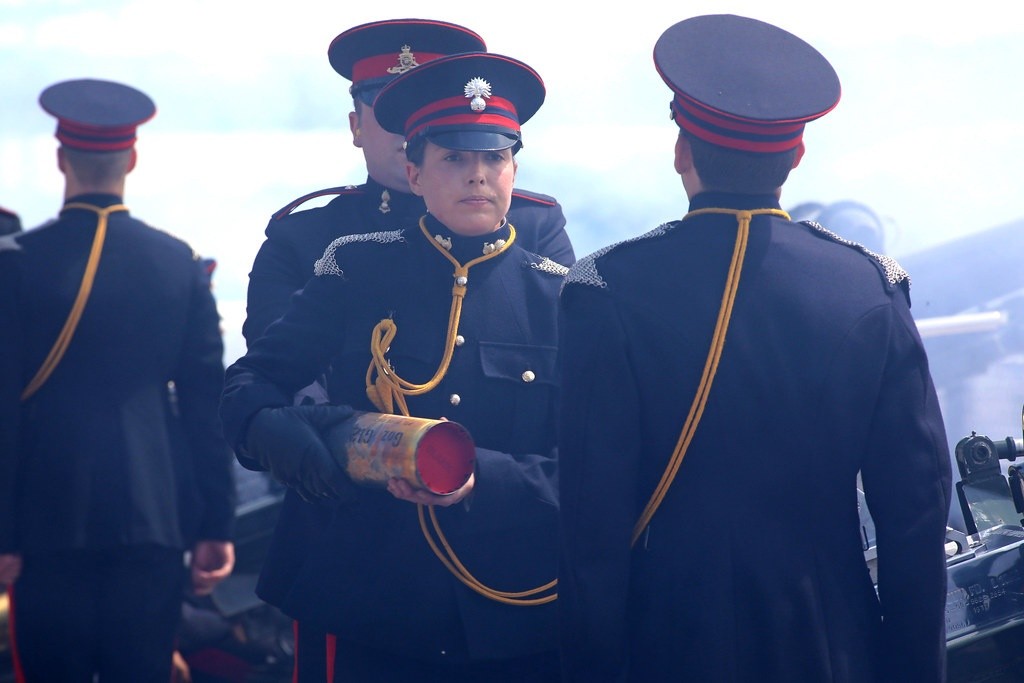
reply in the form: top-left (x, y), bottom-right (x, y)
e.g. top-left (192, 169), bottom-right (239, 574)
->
top-left (328, 19), bottom-right (487, 108)
top-left (372, 51), bottom-right (546, 152)
top-left (41, 79), bottom-right (155, 151)
top-left (653, 14), bottom-right (841, 155)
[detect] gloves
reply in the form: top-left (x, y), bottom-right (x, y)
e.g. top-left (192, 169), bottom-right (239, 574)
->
top-left (243, 405), bottom-right (353, 513)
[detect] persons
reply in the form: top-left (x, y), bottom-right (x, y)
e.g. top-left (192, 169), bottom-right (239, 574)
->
top-left (217, 88), bottom-right (569, 683)
top-left (243, 44), bottom-right (580, 353)
top-left (0, 125), bottom-right (242, 683)
top-left (560, 57), bottom-right (954, 683)
